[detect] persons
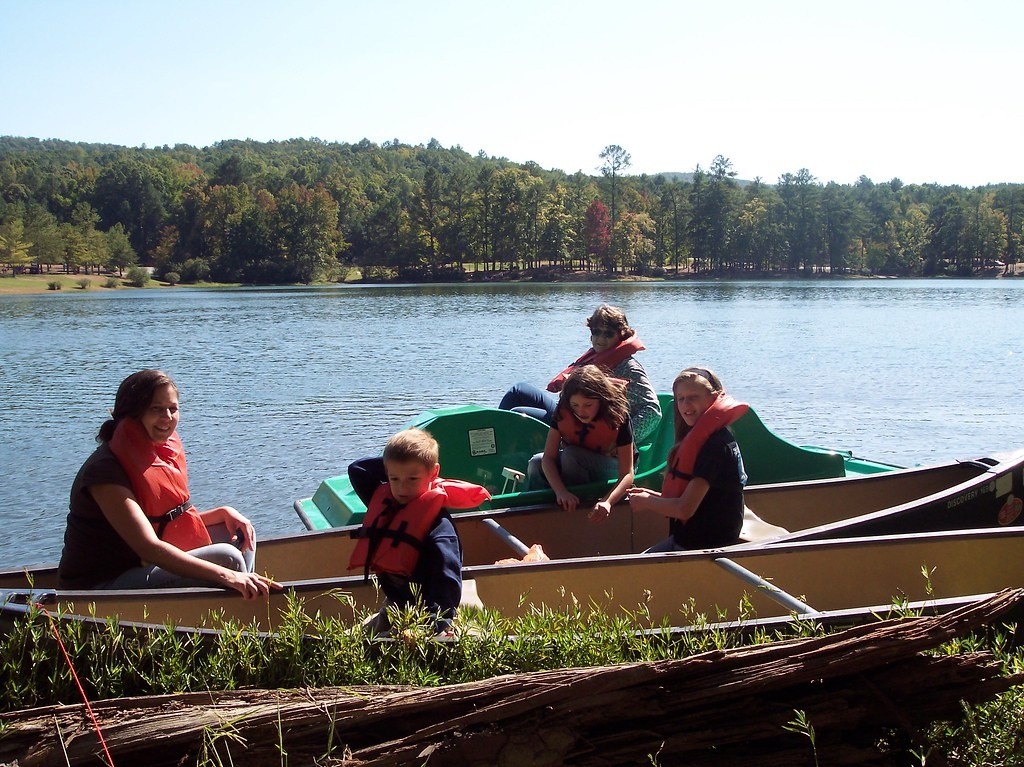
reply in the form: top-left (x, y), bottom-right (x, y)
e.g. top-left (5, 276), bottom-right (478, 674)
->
top-left (520, 365), bottom-right (641, 522)
top-left (55, 372), bottom-right (282, 600)
top-left (354, 426), bottom-right (496, 684)
top-left (495, 304), bottom-right (662, 467)
top-left (624, 366), bottom-right (752, 558)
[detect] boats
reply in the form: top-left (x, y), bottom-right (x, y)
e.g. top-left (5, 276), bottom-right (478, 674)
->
top-left (0, 527), bottom-right (1024, 716)
top-left (294, 391), bottom-right (920, 535)
top-left (2, 446), bottom-right (1024, 591)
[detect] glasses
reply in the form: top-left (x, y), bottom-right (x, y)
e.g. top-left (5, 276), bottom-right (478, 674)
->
top-left (591, 328), bottom-right (618, 339)
